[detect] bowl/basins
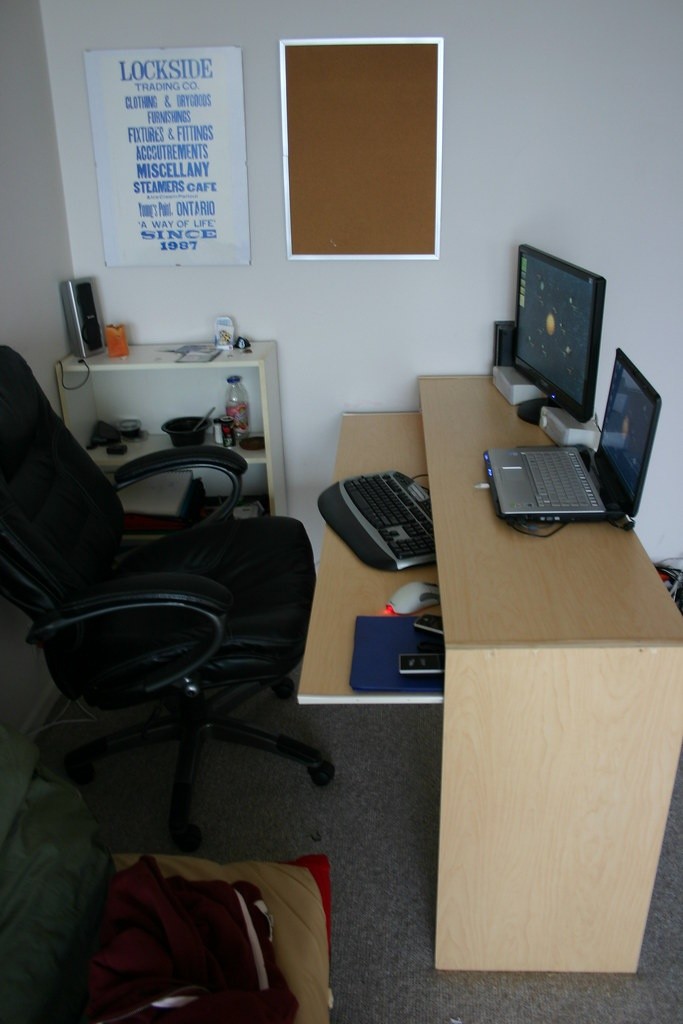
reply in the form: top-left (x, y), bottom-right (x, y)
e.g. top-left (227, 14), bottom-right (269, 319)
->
top-left (161, 416), bottom-right (211, 447)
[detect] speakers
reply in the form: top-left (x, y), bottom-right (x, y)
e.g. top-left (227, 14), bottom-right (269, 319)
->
top-left (60, 276), bottom-right (105, 358)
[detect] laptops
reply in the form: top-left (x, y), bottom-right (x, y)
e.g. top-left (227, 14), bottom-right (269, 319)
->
top-left (483, 347), bottom-right (662, 522)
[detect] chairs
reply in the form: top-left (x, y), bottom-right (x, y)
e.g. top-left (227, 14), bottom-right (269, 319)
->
top-left (0, 344), bottom-right (337, 854)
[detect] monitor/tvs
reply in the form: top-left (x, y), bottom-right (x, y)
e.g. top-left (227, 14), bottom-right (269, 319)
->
top-left (511, 244), bottom-right (606, 426)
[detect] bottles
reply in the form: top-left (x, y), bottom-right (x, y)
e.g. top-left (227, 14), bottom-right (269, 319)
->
top-left (214, 419), bottom-right (223, 443)
top-left (225, 375), bottom-right (250, 441)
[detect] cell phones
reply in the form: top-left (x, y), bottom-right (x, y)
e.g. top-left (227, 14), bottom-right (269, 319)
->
top-left (398, 653), bottom-right (443, 674)
top-left (413, 613), bottom-right (443, 636)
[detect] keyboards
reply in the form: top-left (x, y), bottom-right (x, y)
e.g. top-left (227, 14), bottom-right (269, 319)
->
top-left (317, 470), bottom-right (436, 573)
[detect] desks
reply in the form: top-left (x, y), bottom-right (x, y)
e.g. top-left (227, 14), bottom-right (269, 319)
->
top-left (297, 374), bottom-right (683, 974)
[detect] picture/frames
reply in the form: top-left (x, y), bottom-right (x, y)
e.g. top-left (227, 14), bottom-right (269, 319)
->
top-left (279, 37), bottom-right (444, 260)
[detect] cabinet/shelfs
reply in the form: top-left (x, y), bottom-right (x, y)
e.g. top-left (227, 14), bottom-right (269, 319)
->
top-left (55, 340), bottom-right (288, 541)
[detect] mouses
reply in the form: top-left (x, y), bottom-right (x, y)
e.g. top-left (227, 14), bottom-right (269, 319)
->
top-left (387, 582), bottom-right (440, 614)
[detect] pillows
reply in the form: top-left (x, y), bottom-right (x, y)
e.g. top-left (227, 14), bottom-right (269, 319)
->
top-left (114, 851), bottom-right (332, 1024)
